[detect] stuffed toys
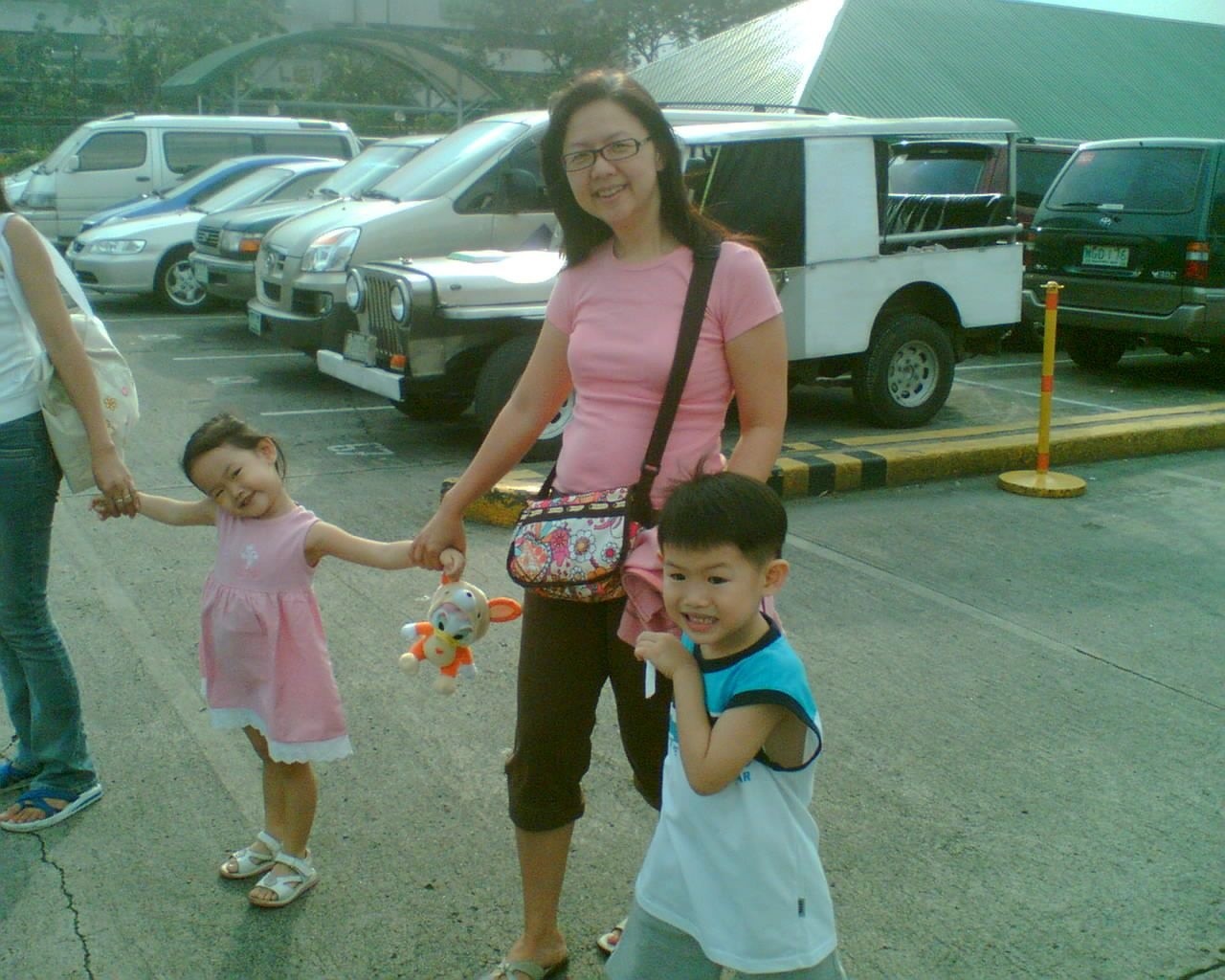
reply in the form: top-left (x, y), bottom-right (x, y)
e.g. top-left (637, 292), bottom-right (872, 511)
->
top-left (398, 573), bottom-right (522, 695)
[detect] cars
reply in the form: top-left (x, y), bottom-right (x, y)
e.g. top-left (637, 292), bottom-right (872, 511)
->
top-left (3, 97), bottom-right (1027, 465)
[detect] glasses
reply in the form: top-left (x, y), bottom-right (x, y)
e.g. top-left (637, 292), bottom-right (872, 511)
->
top-left (557, 136), bottom-right (656, 171)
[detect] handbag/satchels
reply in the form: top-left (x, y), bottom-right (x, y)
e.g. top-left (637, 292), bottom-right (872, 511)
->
top-left (0, 213), bottom-right (141, 492)
top-left (507, 481), bottom-right (655, 603)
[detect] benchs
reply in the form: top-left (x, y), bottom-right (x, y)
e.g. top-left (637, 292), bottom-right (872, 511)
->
top-left (881, 192), bottom-right (1014, 249)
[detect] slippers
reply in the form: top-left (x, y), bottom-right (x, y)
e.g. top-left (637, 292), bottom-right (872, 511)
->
top-left (595, 917), bottom-right (629, 955)
top-left (0, 759), bottom-right (37, 793)
top-left (0, 782), bottom-right (105, 832)
top-left (478, 953), bottom-right (569, 979)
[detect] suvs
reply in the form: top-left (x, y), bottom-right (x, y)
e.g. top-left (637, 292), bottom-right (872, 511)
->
top-left (886, 138), bottom-right (1096, 352)
top-left (1020, 137), bottom-right (1225, 371)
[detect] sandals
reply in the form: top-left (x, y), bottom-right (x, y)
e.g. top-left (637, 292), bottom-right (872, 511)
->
top-left (249, 848), bottom-right (317, 905)
top-left (218, 832), bottom-right (285, 879)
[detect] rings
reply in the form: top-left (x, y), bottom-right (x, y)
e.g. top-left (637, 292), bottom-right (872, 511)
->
top-left (130, 487), bottom-right (137, 492)
top-left (124, 494), bottom-right (131, 498)
top-left (115, 498), bottom-right (123, 503)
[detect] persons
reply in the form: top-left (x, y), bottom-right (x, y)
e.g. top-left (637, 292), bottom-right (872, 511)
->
top-left (409, 68), bottom-right (788, 980)
top-left (0, 183), bottom-right (138, 832)
top-left (88, 413), bottom-right (467, 908)
top-left (603, 475), bottom-right (848, 980)
